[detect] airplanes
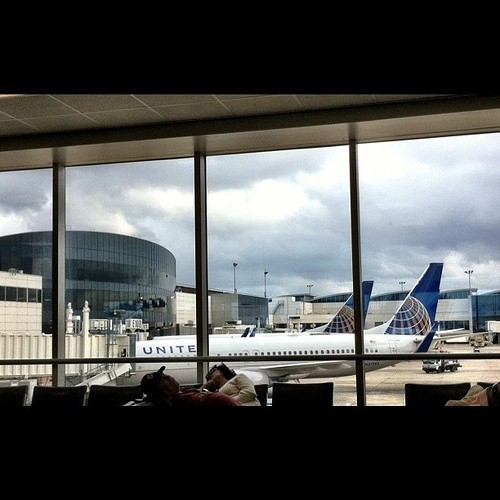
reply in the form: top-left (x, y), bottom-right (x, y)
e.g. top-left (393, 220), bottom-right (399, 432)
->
top-left (135, 263), bottom-right (443, 400)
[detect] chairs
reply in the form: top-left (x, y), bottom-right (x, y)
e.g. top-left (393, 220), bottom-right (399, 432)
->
top-left (272, 383), bottom-right (334, 405)
top-left (406, 384), bottom-right (471, 406)
top-left (0, 386), bottom-right (143, 407)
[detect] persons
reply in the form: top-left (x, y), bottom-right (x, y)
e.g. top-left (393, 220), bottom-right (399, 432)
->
top-left (198, 365), bottom-right (262, 407)
top-left (141, 368), bottom-right (241, 408)
top-left (445, 381), bottom-right (500, 406)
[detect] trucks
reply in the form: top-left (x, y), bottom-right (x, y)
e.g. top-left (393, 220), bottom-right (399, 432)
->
top-left (422, 359), bottom-right (462, 373)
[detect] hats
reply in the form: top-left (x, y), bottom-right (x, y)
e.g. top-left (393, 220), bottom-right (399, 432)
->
top-left (140, 366), bottom-right (165, 396)
